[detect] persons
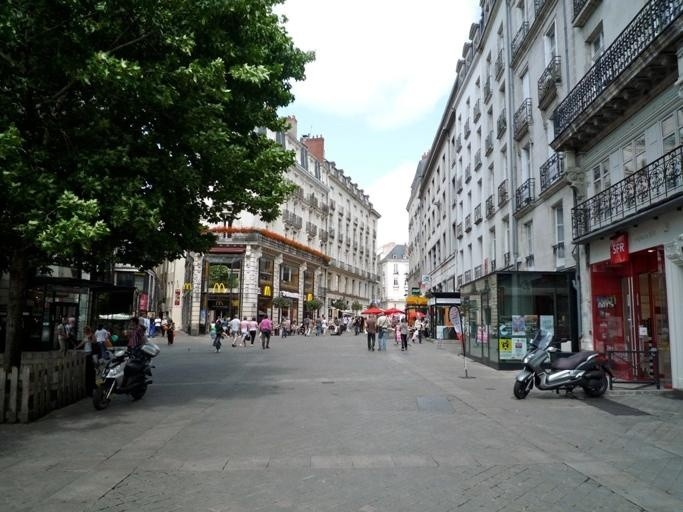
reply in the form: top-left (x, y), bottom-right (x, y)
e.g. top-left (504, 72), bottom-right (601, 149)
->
top-left (73, 326), bottom-right (98, 357)
top-left (211, 313), bottom-right (430, 352)
top-left (65, 317), bottom-right (76, 350)
top-left (95, 325), bottom-right (108, 357)
top-left (57, 318), bottom-right (66, 355)
top-left (124, 310), bottom-right (173, 348)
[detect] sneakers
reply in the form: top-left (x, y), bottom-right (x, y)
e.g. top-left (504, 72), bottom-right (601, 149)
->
top-left (401, 346), bottom-right (409, 352)
top-left (368, 346), bottom-right (387, 353)
top-left (215, 344), bottom-right (270, 354)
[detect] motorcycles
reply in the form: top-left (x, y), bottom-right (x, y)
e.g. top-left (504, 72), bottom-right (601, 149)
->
top-left (90, 332), bottom-right (161, 411)
top-left (513, 324), bottom-right (614, 400)
